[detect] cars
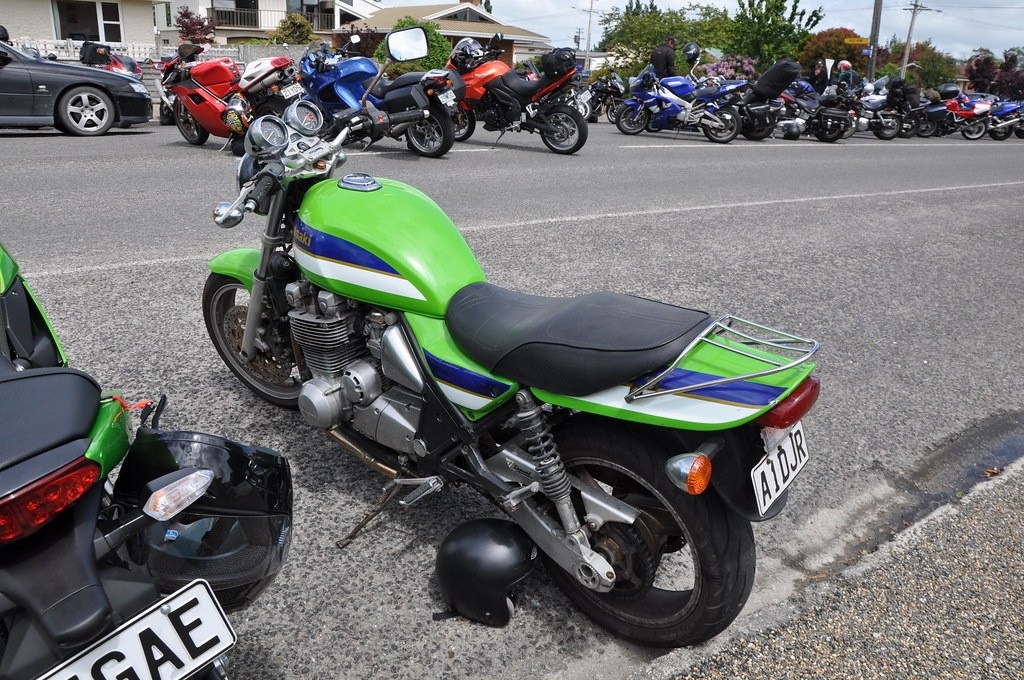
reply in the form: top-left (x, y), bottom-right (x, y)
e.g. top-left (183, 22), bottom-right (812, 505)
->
top-left (0, 41), bottom-right (153, 135)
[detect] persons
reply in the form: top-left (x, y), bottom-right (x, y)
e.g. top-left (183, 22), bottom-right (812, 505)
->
top-left (650, 37), bottom-right (679, 79)
top-left (837, 61), bottom-right (863, 94)
top-left (810, 59), bottom-right (828, 94)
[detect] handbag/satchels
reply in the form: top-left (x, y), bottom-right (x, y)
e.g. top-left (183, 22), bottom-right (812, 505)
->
top-left (80, 42), bottom-right (110, 66)
top-left (885, 76), bottom-right (905, 103)
top-left (752, 57), bottom-right (802, 100)
top-left (541, 47), bottom-right (577, 80)
top-left (923, 87), bottom-right (941, 102)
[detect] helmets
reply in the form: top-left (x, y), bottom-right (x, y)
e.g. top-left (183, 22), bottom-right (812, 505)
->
top-left (683, 42), bottom-right (700, 64)
top-left (106, 396), bottom-right (293, 616)
top-left (837, 60), bottom-right (852, 72)
top-left (433, 518), bottom-right (542, 628)
top-left (0, 25), bottom-right (9, 42)
top-left (782, 123), bottom-right (800, 140)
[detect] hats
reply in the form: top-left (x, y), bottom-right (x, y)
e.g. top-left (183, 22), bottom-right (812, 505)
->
top-left (815, 61), bottom-right (824, 66)
top-left (664, 36), bottom-right (676, 46)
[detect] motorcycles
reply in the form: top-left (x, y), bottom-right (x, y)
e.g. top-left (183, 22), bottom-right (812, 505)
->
top-left (1, 245), bottom-right (295, 680)
top-left (615, 50), bottom-right (1024, 143)
top-left (199, 27), bottom-right (820, 645)
top-left (79, 42), bottom-right (150, 81)
top-left (143, 43), bottom-right (308, 147)
top-left (296, 33), bottom-right (458, 157)
top-left (516, 57), bottom-right (627, 124)
top-left (444, 32), bottom-right (589, 154)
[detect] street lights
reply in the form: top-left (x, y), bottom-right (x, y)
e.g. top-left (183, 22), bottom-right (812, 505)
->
top-left (901, 9), bottom-right (942, 79)
